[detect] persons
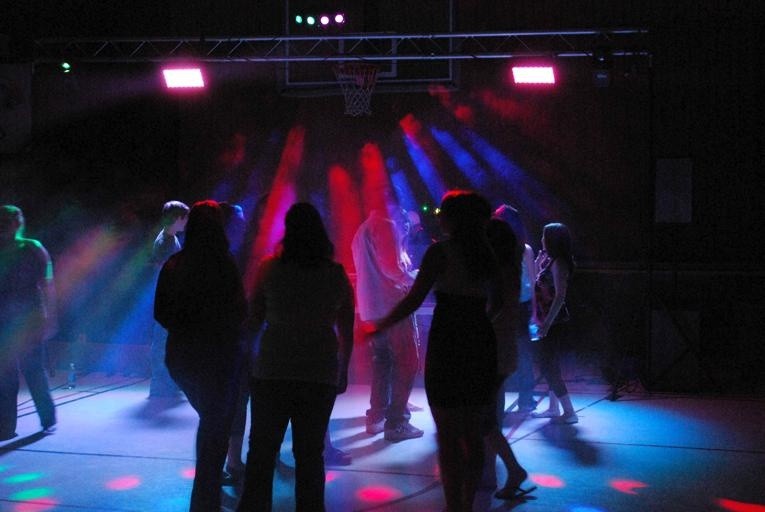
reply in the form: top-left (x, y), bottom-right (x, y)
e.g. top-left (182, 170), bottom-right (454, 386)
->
top-left (0, 204), bottom-right (57, 441)
top-left (350, 181), bottom-right (579, 441)
top-left (362, 190), bottom-right (528, 512)
top-left (148, 190), bottom-right (354, 512)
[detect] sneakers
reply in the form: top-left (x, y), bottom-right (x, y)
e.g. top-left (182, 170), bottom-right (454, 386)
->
top-left (383, 422), bottom-right (424, 442)
top-left (42, 426), bottom-right (57, 433)
top-left (322, 448), bottom-right (352, 466)
top-left (221, 495), bottom-right (241, 512)
top-left (149, 379), bottom-right (183, 398)
top-left (365, 416), bottom-right (388, 435)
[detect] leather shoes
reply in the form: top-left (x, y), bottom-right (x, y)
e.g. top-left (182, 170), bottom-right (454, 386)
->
top-left (538, 408), bottom-right (561, 417)
top-left (550, 415), bottom-right (578, 424)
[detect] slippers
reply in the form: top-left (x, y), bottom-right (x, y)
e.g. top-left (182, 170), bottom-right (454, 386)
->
top-left (494, 485), bottom-right (537, 500)
